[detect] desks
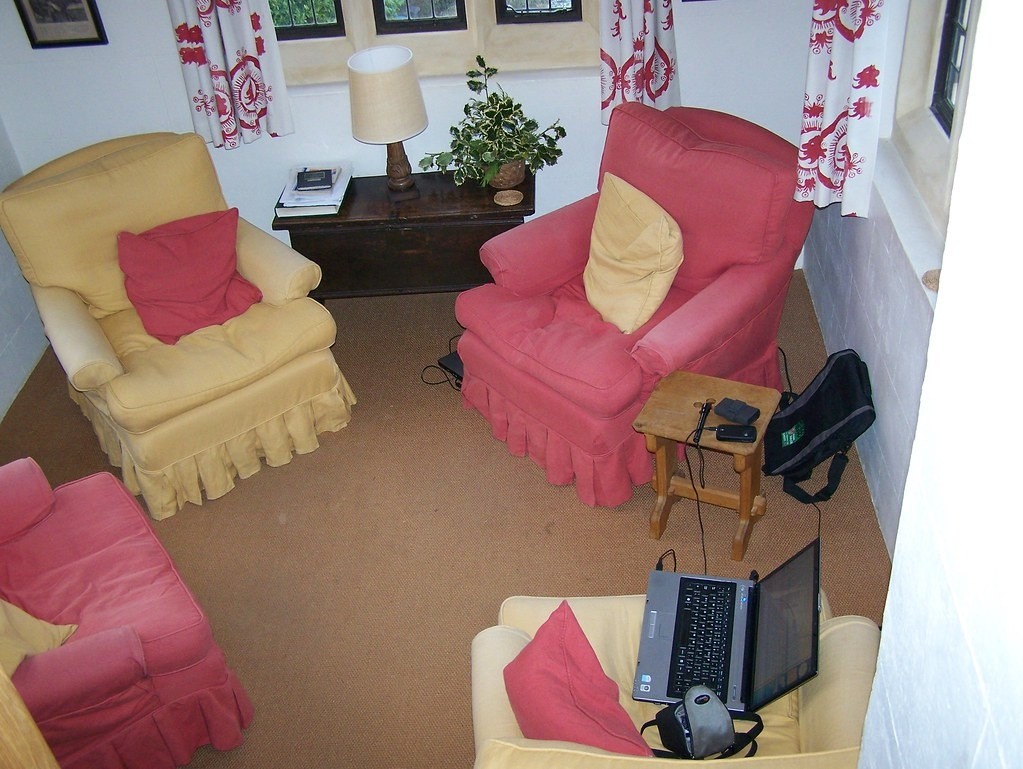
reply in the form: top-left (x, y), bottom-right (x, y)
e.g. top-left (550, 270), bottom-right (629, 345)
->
top-left (272, 164), bottom-right (535, 306)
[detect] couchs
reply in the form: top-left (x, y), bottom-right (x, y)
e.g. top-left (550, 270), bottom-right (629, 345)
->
top-left (454, 100), bottom-right (814, 509)
top-left (0, 456), bottom-right (256, 769)
top-left (0, 132), bottom-right (357, 522)
top-left (470, 594), bottom-right (880, 769)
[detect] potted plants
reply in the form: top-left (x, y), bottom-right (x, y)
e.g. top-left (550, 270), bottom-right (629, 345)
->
top-left (418, 55), bottom-right (567, 189)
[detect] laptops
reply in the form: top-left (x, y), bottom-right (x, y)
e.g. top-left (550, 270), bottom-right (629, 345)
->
top-left (438, 349), bottom-right (464, 382)
top-left (633, 536), bottom-right (821, 714)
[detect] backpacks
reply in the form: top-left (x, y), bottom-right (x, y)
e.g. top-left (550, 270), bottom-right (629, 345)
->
top-left (762, 350), bottom-right (877, 474)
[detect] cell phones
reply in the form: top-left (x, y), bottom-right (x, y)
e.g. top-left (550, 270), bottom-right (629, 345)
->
top-left (716, 424), bottom-right (757, 443)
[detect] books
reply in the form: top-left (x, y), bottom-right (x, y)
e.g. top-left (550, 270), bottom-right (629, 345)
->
top-left (274, 166), bottom-right (349, 220)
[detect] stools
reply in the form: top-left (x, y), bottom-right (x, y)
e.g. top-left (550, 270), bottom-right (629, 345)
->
top-left (632, 371), bottom-right (783, 562)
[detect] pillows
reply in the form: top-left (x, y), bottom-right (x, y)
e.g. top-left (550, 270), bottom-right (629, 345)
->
top-left (582, 170), bottom-right (684, 335)
top-left (117, 205), bottom-right (264, 346)
top-left (503, 600), bottom-right (656, 757)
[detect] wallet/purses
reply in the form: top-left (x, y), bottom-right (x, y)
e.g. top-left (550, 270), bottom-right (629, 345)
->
top-left (714, 398), bottom-right (760, 424)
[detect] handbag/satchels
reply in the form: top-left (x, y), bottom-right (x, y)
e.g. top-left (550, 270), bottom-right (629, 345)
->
top-left (640, 685), bottom-right (765, 759)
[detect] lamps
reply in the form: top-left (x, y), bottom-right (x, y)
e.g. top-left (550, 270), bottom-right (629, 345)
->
top-left (346, 45), bottom-right (429, 193)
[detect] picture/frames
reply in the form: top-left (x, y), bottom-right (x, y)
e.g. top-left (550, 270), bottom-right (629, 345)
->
top-left (14, 0), bottom-right (109, 50)
